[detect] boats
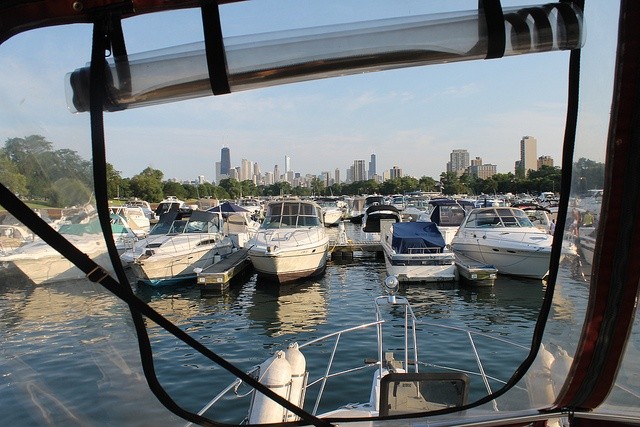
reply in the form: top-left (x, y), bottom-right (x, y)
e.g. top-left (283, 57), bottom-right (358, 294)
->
top-left (386, 194), bottom-right (444, 220)
top-left (361, 204), bottom-right (403, 252)
top-left (324, 208), bottom-right (343, 225)
top-left (119, 200), bottom-right (260, 287)
top-left (351, 199), bottom-right (365, 223)
top-left (523, 205), bottom-right (552, 234)
top-left (363, 195), bottom-right (386, 217)
top-left (458, 192), bottom-right (559, 211)
top-left (381, 219), bottom-right (456, 283)
top-left (428, 204), bottom-right (469, 248)
top-left (0, 225), bottom-right (38, 276)
top-left (451, 207), bottom-right (580, 280)
top-left (416, 198), bottom-right (478, 246)
top-left (244, 201), bottom-right (329, 283)
top-left (0, 214), bottom-right (143, 285)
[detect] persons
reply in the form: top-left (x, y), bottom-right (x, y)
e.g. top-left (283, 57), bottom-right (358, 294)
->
top-left (549, 219), bottom-right (556, 236)
top-left (582, 211), bottom-right (594, 227)
